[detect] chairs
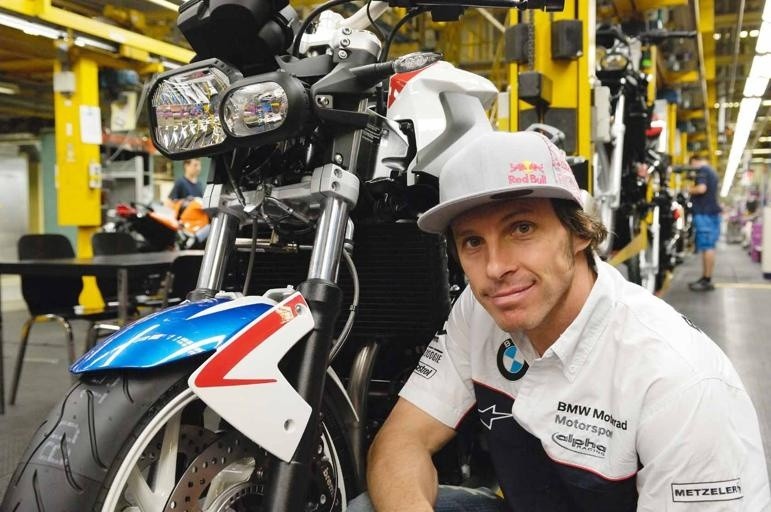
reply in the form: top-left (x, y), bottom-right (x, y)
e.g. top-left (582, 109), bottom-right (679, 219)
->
top-left (7, 227), bottom-right (205, 405)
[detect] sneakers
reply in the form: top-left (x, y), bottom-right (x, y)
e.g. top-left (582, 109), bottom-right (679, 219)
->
top-left (688, 277), bottom-right (714, 291)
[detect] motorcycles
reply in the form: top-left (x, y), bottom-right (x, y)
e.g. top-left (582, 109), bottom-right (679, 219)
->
top-left (594, 23), bottom-right (706, 295)
top-left (0, 0), bottom-right (565, 512)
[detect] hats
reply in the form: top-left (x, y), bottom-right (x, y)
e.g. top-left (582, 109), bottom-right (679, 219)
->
top-left (417, 130), bottom-right (586, 237)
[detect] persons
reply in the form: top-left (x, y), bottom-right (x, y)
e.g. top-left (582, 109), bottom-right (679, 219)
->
top-left (680, 155), bottom-right (722, 292)
top-left (346, 129), bottom-right (770, 511)
top-left (162, 157), bottom-right (204, 222)
top-left (745, 190), bottom-right (760, 224)
